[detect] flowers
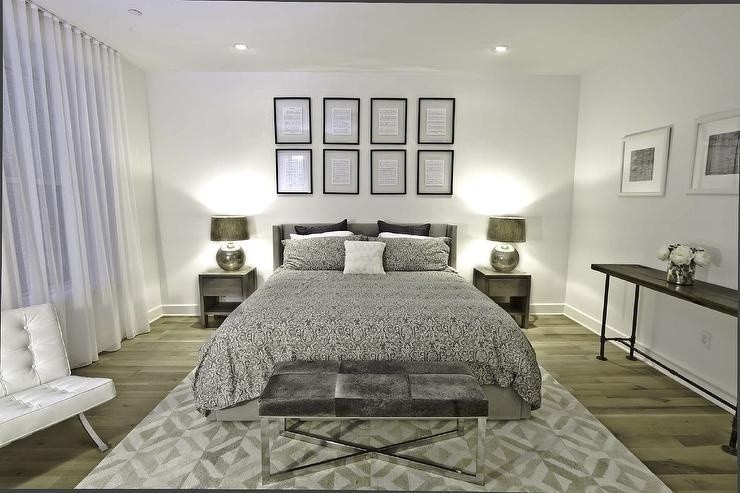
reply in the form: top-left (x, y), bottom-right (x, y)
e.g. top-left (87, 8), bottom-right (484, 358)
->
top-left (656, 240), bottom-right (712, 267)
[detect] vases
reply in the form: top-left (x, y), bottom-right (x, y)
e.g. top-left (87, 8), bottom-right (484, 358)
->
top-left (666, 263), bottom-right (696, 285)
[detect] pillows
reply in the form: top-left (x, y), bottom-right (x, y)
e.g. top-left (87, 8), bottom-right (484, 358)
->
top-left (280, 219), bottom-right (453, 275)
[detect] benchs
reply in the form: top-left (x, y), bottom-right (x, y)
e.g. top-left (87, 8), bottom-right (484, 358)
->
top-left (0, 303), bottom-right (117, 453)
top-left (257, 361), bottom-right (489, 486)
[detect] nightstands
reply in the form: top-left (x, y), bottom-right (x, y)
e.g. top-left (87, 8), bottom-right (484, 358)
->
top-left (473, 267), bottom-right (531, 329)
top-left (199, 268), bottom-right (257, 328)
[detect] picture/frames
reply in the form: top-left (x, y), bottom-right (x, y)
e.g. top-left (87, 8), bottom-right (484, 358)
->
top-left (619, 125), bottom-right (671, 197)
top-left (685, 108), bottom-right (739, 195)
top-left (273, 96), bottom-right (456, 195)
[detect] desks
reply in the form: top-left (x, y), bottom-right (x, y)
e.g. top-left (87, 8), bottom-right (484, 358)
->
top-left (591, 264), bottom-right (740, 456)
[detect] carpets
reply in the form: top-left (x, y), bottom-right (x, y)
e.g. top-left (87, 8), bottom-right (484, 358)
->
top-left (73, 366), bottom-right (675, 493)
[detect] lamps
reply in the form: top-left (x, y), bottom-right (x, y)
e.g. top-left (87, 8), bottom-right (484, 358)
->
top-left (487, 216), bottom-right (526, 273)
top-left (210, 215), bottom-right (250, 271)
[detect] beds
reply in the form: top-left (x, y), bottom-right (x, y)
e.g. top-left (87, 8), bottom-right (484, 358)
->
top-left (205, 224), bottom-right (531, 421)
